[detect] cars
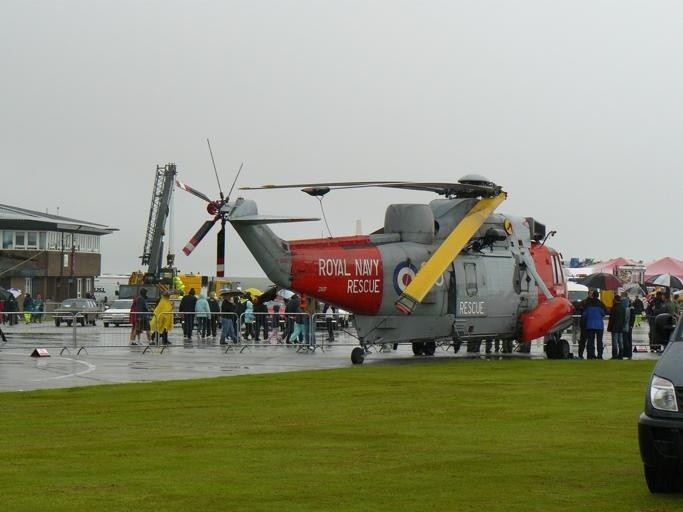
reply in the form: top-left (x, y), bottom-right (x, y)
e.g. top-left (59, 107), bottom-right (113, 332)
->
top-left (52, 298), bottom-right (99, 326)
top-left (100, 298), bottom-right (134, 328)
top-left (252, 295), bottom-right (349, 331)
top-left (636, 308), bottom-right (682, 494)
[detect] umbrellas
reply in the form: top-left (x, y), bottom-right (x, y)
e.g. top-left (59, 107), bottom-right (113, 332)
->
top-left (579, 272), bottom-right (623, 291)
top-left (644, 273), bottom-right (682, 291)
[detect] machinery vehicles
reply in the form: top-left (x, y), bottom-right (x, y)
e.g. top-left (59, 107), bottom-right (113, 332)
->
top-left (115, 163), bottom-right (240, 329)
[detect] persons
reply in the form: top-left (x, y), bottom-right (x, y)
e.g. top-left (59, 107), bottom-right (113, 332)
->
top-left (578, 290), bottom-right (682, 359)
top-left (128, 286), bottom-right (333, 347)
top-left (0, 287), bottom-right (46, 340)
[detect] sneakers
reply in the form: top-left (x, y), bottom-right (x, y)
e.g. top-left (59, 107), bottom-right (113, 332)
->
top-left (219, 341), bottom-right (228, 344)
top-left (0, 320), bottom-right (42, 342)
top-left (148, 341), bottom-right (155, 345)
top-left (233, 338), bottom-right (243, 343)
top-left (129, 341), bottom-right (143, 345)
top-left (197, 332), bottom-right (217, 339)
top-left (244, 335), bottom-right (316, 350)
top-left (183, 335), bottom-right (192, 340)
top-left (577, 324), bottom-right (670, 360)
top-left (162, 340), bottom-right (172, 345)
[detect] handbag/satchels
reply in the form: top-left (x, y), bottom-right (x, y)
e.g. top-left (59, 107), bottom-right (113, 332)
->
top-left (325, 306), bottom-right (335, 319)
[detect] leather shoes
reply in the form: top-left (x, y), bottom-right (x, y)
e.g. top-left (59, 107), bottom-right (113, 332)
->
top-left (326, 335), bottom-right (335, 342)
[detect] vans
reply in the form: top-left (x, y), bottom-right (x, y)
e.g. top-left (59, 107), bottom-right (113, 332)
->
top-left (565, 281), bottom-right (589, 324)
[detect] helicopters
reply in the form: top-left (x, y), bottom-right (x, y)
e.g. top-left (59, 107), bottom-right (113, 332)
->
top-left (173, 137), bottom-right (576, 365)
